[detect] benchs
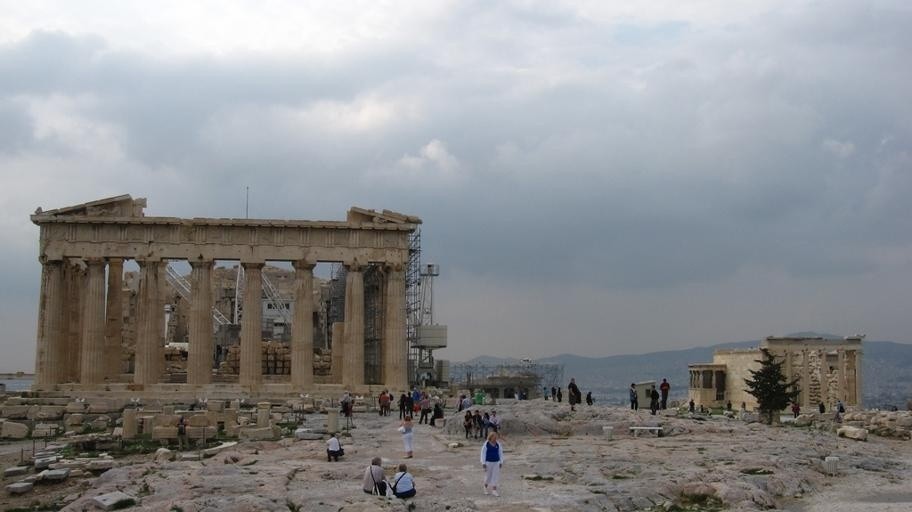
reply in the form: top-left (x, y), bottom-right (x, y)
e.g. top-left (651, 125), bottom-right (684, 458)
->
top-left (629, 426), bottom-right (664, 438)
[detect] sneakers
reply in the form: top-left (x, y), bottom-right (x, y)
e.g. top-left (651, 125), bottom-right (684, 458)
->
top-left (491, 490), bottom-right (500, 497)
top-left (484, 486), bottom-right (489, 496)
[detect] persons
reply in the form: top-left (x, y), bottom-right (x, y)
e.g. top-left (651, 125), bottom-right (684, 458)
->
top-left (792, 404), bottom-right (800, 418)
top-left (819, 401), bottom-right (825, 413)
top-left (480, 432), bottom-right (504, 497)
top-left (543, 377), bottom-right (597, 411)
top-left (480, 412), bottom-right (490, 439)
top-left (688, 398), bottom-right (695, 412)
top-left (326, 433), bottom-right (340, 461)
top-left (399, 416), bottom-right (415, 459)
top-left (472, 409), bottom-right (484, 439)
top-left (177, 415), bottom-right (190, 451)
top-left (659, 378), bottom-right (671, 409)
top-left (462, 409), bottom-right (473, 439)
top-left (339, 384), bottom-right (474, 432)
top-left (726, 399), bottom-right (732, 411)
top-left (363, 456), bottom-right (394, 494)
top-left (489, 410), bottom-right (502, 433)
top-left (629, 382), bottom-right (638, 411)
top-left (649, 384), bottom-right (659, 415)
top-left (394, 463), bottom-right (417, 498)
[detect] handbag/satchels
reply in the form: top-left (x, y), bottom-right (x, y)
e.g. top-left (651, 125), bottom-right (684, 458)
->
top-left (336, 435), bottom-right (344, 457)
top-left (398, 426), bottom-right (406, 433)
top-left (393, 472), bottom-right (407, 495)
top-left (370, 466), bottom-right (389, 496)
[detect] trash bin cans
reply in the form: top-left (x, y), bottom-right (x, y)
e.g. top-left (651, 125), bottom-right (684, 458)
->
top-left (475, 392), bottom-right (484, 405)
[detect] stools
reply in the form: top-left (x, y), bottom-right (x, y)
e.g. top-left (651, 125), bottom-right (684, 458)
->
top-left (602, 426), bottom-right (614, 440)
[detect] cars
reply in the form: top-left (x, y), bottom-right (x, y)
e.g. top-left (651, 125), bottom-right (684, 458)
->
top-left (877, 404), bottom-right (897, 412)
